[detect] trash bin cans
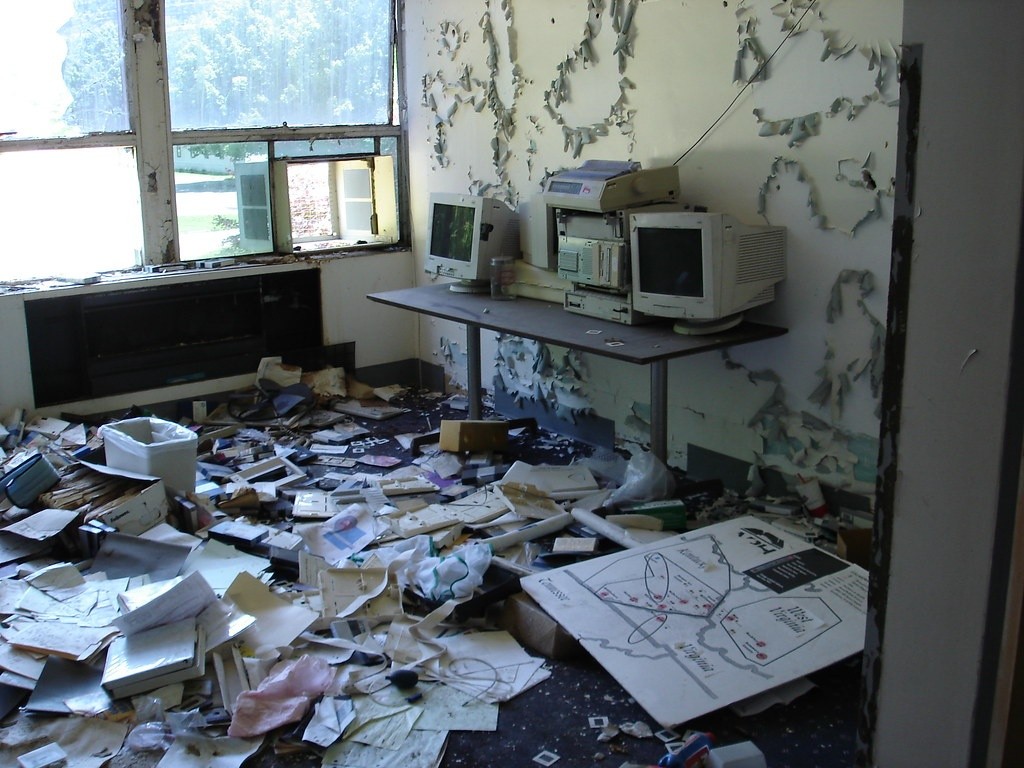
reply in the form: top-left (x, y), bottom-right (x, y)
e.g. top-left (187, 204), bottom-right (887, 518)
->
top-left (101, 417), bottom-right (198, 494)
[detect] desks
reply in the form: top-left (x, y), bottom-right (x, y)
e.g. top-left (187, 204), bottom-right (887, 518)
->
top-left (365, 281), bottom-right (789, 467)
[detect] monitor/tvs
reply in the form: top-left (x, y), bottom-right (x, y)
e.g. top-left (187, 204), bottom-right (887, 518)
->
top-left (629, 208), bottom-right (788, 336)
top-left (423, 192), bottom-right (524, 295)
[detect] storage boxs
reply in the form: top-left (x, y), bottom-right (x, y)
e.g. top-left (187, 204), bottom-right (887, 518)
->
top-left (499, 593), bottom-right (578, 660)
top-left (708, 740), bottom-right (767, 768)
top-left (439, 419), bottom-right (509, 452)
top-left (619, 499), bottom-right (687, 531)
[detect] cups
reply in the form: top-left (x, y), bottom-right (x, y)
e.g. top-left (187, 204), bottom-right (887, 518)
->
top-left (795, 476), bottom-right (827, 518)
